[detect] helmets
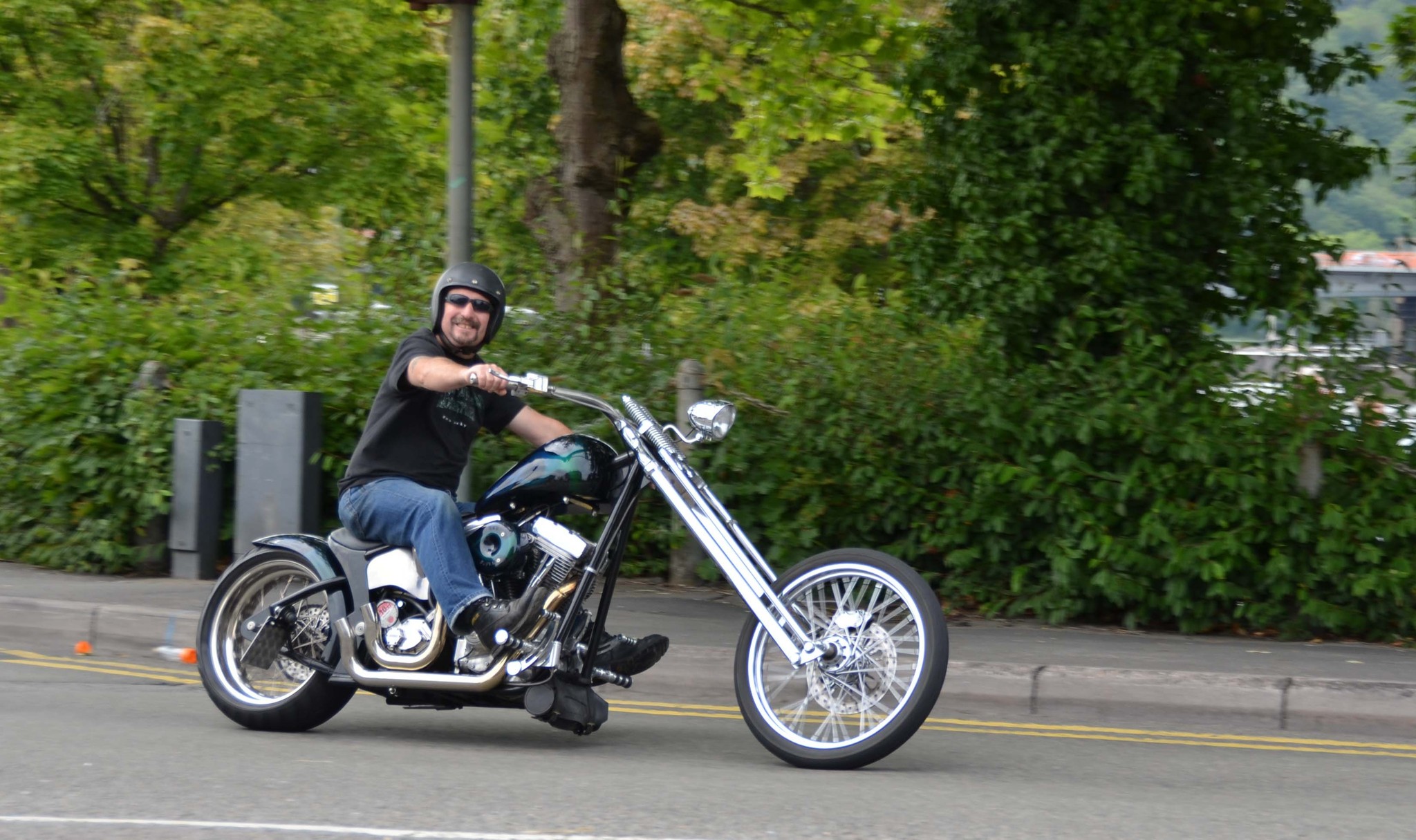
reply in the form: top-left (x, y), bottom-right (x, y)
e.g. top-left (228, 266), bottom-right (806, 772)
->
top-left (432, 263), bottom-right (506, 343)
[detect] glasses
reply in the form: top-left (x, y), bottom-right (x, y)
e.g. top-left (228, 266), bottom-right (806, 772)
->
top-left (442, 294), bottom-right (492, 312)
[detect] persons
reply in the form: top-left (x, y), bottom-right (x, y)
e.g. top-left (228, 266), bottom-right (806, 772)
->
top-left (338, 263), bottom-right (670, 687)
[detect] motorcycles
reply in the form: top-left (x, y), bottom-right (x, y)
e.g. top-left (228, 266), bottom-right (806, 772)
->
top-left (193, 369), bottom-right (952, 773)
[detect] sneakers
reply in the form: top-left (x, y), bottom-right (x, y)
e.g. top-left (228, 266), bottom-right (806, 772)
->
top-left (466, 587), bottom-right (548, 658)
top-left (590, 632), bottom-right (669, 686)
